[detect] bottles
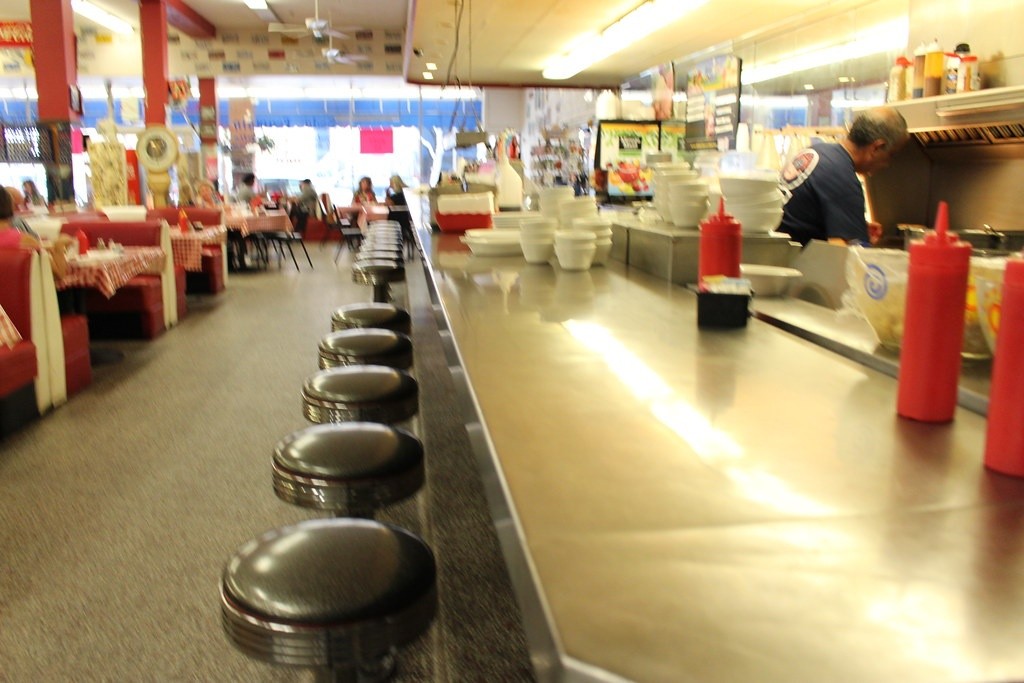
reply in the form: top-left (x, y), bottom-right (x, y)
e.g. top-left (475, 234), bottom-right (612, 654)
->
top-left (978, 255), bottom-right (1024, 478)
top-left (887, 35), bottom-right (982, 104)
top-left (892, 198), bottom-right (964, 424)
top-left (696, 195), bottom-right (740, 282)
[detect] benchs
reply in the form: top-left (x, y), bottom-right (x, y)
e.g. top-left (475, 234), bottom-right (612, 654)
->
top-left (162, 220), bottom-right (188, 326)
top-left (35, 245), bottom-right (92, 407)
top-left (57, 219), bottom-right (166, 338)
top-left (146, 206), bottom-right (225, 294)
top-left (0, 247), bottom-right (52, 416)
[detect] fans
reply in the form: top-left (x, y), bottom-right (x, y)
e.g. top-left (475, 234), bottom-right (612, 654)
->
top-left (268, 1), bottom-right (363, 43)
top-left (316, 12), bottom-right (369, 68)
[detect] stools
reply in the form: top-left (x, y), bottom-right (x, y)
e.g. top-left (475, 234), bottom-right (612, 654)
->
top-left (317, 327), bottom-right (414, 370)
top-left (331, 302), bottom-right (412, 335)
top-left (352, 219), bottom-right (405, 302)
top-left (271, 422), bottom-right (426, 522)
top-left (216, 517), bottom-right (440, 683)
top-left (300, 363), bottom-right (421, 427)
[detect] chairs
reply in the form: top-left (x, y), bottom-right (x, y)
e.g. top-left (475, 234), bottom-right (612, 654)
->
top-left (332, 204), bottom-right (363, 262)
top-left (316, 195), bottom-right (351, 243)
top-left (226, 188), bottom-right (313, 270)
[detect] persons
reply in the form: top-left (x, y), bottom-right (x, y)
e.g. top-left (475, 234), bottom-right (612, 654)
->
top-left (177, 169), bottom-right (412, 276)
top-left (770, 104), bottom-right (910, 250)
top-left (0, 180), bottom-right (75, 282)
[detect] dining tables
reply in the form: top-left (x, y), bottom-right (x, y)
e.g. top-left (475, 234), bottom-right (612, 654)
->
top-left (56, 245), bottom-right (163, 312)
top-left (337, 205), bottom-right (410, 215)
top-left (169, 224), bottom-right (226, 241)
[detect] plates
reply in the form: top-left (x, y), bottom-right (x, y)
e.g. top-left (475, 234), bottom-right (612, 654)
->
top-left (455, 211), bottom-right (541, 259)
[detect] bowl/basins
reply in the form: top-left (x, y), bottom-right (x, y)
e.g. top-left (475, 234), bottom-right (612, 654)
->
top-left (741, 262), bottom-right (802, 297)
top-left (519, 185), bottom-right (614, 272)
top-left (648, 161), bottom-right (786, 234)
